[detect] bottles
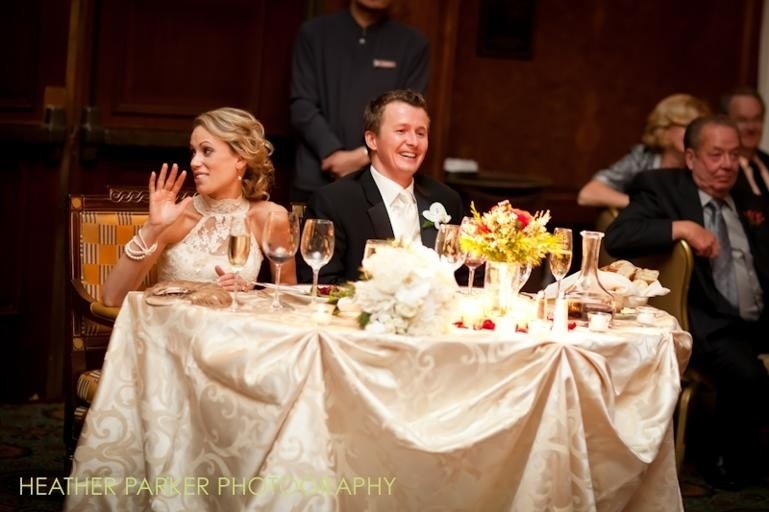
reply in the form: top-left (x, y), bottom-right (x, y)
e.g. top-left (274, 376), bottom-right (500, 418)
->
top-left (553, 229), bottom-right (617, 326)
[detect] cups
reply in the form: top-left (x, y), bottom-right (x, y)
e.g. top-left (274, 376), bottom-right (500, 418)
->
top-left (361, 238), bottom-right (395, 280)
top-left (586, 310), bottom-right (612, 333)
top-left (458, 215), bottom-right (491, 296)
top-left (483, 258), bottom-right (533, 312)
top-left (434, 223), bottom-right (469, 272)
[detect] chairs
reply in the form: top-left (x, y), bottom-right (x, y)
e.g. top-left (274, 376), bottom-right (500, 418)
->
top-left (71, 186), bottom-right (197, 459)
top-left (594, 208), bottom-right (688, 472)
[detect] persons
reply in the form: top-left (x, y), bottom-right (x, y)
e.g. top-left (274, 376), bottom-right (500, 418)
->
top-left (576, 93), bottom-right (712, 208)
top-left (602, 114), bottom-right (769, 493)
top-left (100, 107), bottom-right (298, 308)
top-left (288, 89), bottom-right (465, 285)
top-left (717, 85), bottom-right (769, 267)
top-left (289, 0), bottom-right (432, 223)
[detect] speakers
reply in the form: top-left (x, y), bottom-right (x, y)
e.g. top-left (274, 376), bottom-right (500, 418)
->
top-left (475, 0), bottom-right (538, 60)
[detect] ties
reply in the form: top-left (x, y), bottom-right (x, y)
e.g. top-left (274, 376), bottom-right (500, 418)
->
top-left (400, 189), bottom-right (419, 245)
top-left (707, 198), bottom-right (740, 309)
top-left (748, 159), bottom-right (768, 194)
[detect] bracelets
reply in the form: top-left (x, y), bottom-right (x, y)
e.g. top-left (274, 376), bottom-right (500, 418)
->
top-left (123, 228), bottom-right (158, 261)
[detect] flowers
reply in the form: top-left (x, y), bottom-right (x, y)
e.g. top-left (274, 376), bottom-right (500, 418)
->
top-left (458, 200), bottom-right (567, 269)
top-left (420, 201), bottom-right (452, 230)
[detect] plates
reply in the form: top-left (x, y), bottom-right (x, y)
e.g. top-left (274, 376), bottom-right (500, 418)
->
top-left (282, 284), bottom-right (354, 305)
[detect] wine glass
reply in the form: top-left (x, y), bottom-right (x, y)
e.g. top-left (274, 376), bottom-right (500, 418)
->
top-left (298, 218), bottom-right (338, 316)
top-left (226, 214), bottom-right (252, 314)
top-left (253, 210), bottom-right (300, 316)
top-left (548, 226), bottom-right (569, 322)
